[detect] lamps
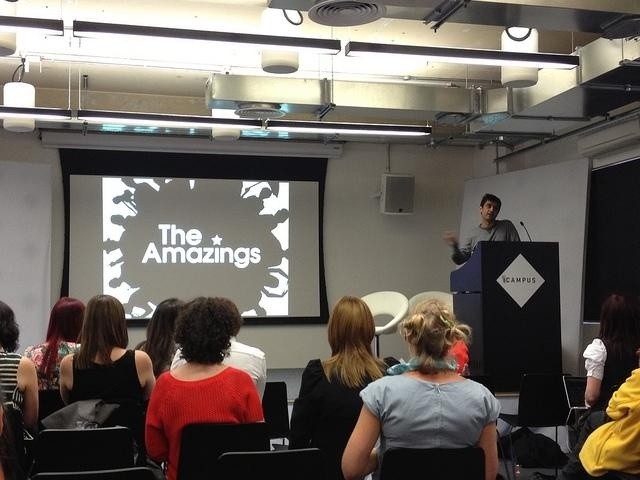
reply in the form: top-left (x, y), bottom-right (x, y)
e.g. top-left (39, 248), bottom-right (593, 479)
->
top-left (3, 56), bottom-right (41, 134)
top-left (500, 25), bottom-right (540, 90)
top-left (212, 68), bottom-right (242, 142)
top-left (260, 8), bottom-right (304, 75)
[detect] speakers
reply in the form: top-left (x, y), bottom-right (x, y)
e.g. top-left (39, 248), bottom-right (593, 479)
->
top-left (380, 173), bottom-right (415, 216)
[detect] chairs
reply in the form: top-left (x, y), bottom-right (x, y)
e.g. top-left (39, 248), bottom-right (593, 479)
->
top-left (32, 467), bottom-right (158, 479)
top-left (261, 381), bottom-right (290, 432)
top-left (31, 425), bottom-right (134, 470)
top-left (24, 385), bottom-right (72, 421)
top-left (377, 447), bottom-right (486, 480)
top-left (499, 371), bottom-right (571, 480)
top-left (212, 447), bottom-right (324, 479)
top-left (175, 420), bottom-right (285, 479)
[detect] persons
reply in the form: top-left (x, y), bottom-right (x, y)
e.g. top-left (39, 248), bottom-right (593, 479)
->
top-left (59, 295), bottom-right (156, 405)
top-left (22, 297), bottom-right (85, 390)
top-left (443, 193), bottom-right (521, 265)
top-left (288, 296), bottom-right (400, 480)
top-left (449, 337), bottom-right (473, 378)
top-left (0, 301), bottom-right (39, 426)
top-left (144, 298), bottom-right (265, 479)
top-left (568, 293), bottom-right (640, 448)
top-left (530, 350), bottom-right (640, 479)
top-left (135, 298), bottom-right (186, 398)
top-left (170, 335), bottom-right (265, 405)
top-left (342, 298), bottom-right (500, 479)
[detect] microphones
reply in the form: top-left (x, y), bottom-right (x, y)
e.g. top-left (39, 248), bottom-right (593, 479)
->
top-left (519, 220), bottom-right (532, 241)
